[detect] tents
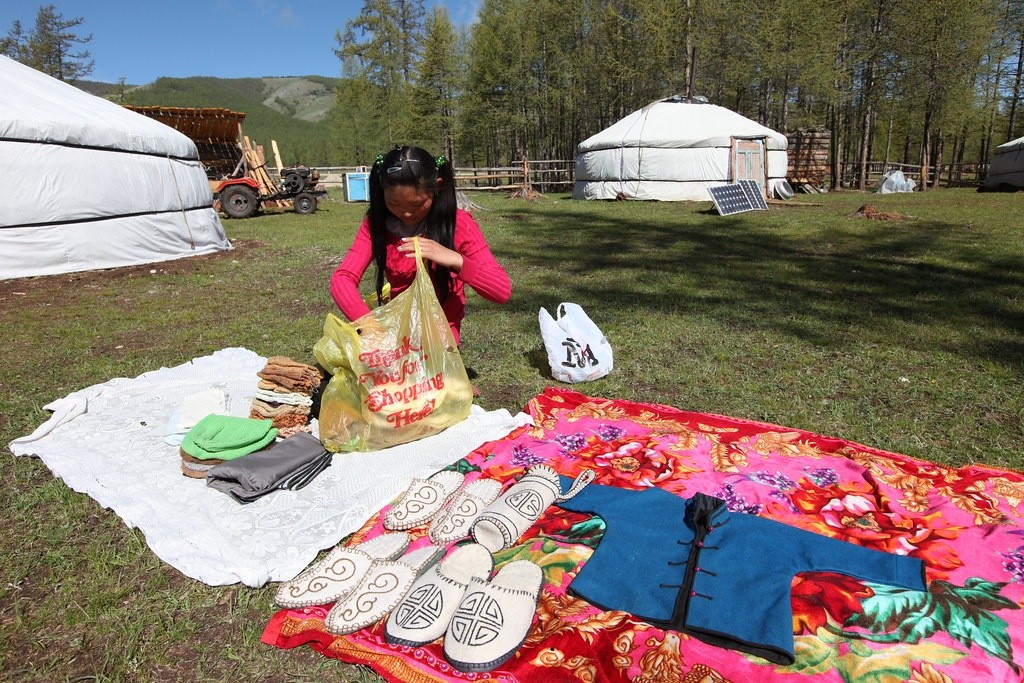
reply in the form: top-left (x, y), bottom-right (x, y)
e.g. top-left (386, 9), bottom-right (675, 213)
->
top-left (0, 50), bottom-right (233, 281)
top-left (574, 96), bottom-right (789, 203)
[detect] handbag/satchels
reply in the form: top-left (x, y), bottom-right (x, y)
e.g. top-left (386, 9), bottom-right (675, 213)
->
top-left (538, 302), bottom-right (613, 384)
top-left (313, 236), bottom-right (472, 455)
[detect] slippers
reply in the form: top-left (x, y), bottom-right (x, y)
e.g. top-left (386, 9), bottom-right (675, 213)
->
top-left (384, 543), bottom-right (495, 647)
top-left (274, 532), bottom-right (410, 609)
top-left (428, 478), bottom-right (503, 545)
top-left (384, 470), bottom-right (465, 530)
top-left (443, 559), bottom-right (544, 672)
top-left (324, 545), bottom-right (446, 635)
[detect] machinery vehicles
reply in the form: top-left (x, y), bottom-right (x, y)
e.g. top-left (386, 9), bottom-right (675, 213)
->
top-left (215, 161), bottom-right (327, 218)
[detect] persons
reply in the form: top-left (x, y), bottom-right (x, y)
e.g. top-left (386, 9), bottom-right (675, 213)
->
top-left (329, 144), bottom-right (512, 346)
